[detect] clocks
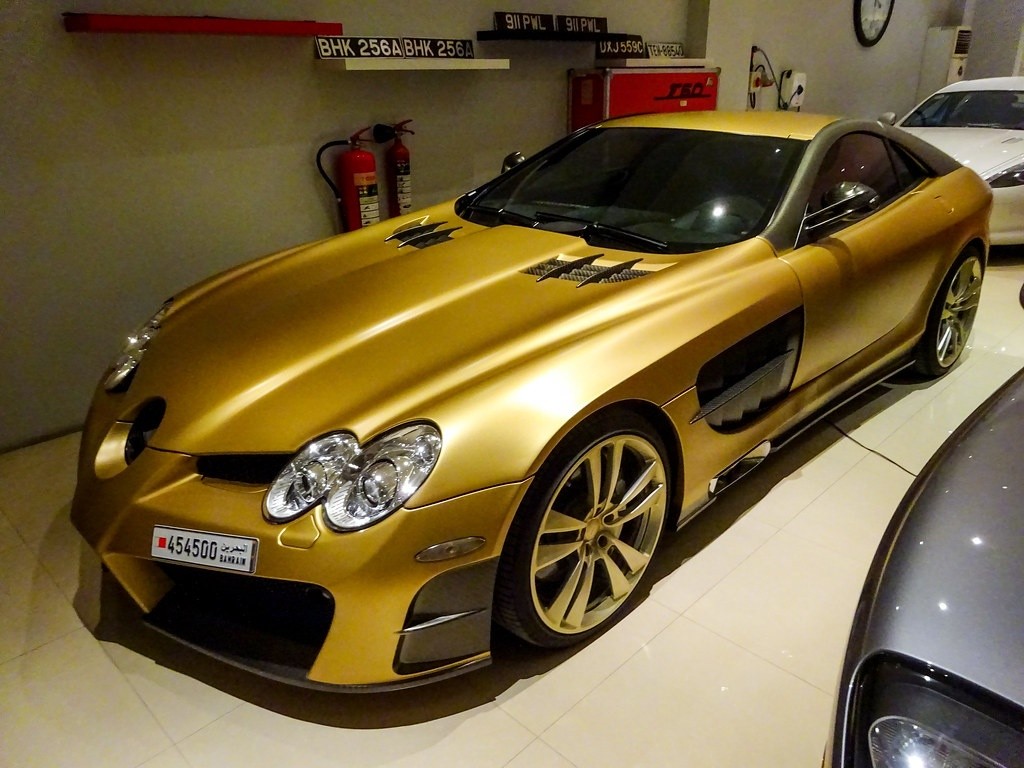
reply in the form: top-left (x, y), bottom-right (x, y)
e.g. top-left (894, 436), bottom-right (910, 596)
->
top-left (854, 0), bottom-right (894, 47)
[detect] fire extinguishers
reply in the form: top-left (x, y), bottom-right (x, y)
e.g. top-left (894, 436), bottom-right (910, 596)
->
top-left (317, 126), bottom-right (380, 232)
top-left (374, 119), bottom-right (415, 217)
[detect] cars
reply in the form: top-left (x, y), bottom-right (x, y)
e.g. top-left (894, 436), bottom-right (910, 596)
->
top-left (880, 75), bottom-right (1024, 243)
top-left (830, 279), bottom-right (1023, 768)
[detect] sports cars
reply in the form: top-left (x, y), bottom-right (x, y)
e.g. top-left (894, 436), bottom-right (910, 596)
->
top-left (66, 111), bottom-right (997, 705)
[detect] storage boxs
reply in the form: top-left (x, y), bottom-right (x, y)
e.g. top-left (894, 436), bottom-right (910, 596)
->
top-left (568, 67), bottom-right (719, 136)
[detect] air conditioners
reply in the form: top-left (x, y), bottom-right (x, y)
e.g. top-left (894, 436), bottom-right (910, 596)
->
top-left (915, 25), bottom-right (972, 109)
